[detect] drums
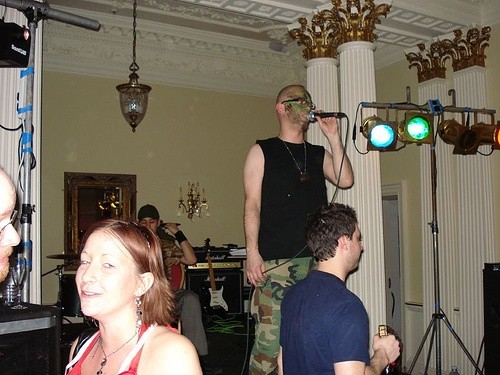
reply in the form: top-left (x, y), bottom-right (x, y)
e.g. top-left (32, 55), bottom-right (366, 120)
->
top-left (60, 270), bottom-right (83, 318)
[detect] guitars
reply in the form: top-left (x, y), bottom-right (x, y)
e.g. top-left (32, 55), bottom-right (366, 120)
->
top-left (203, 236), bottom-right (230, 316)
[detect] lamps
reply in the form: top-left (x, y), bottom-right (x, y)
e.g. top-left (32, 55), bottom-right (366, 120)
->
top-left (177, 179), bottom-right (210, 222)
top-left (472, 120), bottom-right (500, 151)
top-left (437, 119), bottom-right (482, 155)
top-left (397, 112), bottom-right (435, 143)
top-left (362, 116), bottom-right (398, 152)
top-left (116, 0), bottom-right (152, 133)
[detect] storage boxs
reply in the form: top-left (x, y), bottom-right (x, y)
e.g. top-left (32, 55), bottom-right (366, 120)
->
top-left (187, 270), bottom-right (242, 315)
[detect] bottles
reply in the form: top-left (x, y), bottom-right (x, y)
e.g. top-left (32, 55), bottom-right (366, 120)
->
top-left (1, 263), bottom-right (18, 306)
top-left (377, 326), bottom-right (394, 375)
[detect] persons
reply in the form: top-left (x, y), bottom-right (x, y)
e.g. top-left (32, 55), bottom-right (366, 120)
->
top-left (0, 166), bottom-right (22, 283)
top-left (139, 204), bottom-right (220, 371)
top-left (277, 203), bottom-right (400, 375)
top-left (243, 85), bottom-right (355, 375)
top-left (65, 218), bottom-right (204, 375)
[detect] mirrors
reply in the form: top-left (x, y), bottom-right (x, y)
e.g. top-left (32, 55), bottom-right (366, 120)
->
top-left (64, 172), bottom-right (138, 271)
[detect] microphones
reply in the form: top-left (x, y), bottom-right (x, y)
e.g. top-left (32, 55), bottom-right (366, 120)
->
top-left (308, 111), bottom-right (346, 122)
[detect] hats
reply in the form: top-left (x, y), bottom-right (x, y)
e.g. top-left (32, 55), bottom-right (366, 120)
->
top-left (138, 204), bottom-right (160, 221)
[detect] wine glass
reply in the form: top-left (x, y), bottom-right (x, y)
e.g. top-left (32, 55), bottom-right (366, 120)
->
top-left (10, 258), bottom-right (28, 309)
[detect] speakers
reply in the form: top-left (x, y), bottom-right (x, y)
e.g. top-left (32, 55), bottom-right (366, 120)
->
top-left (187, 270), bottom-right (244, 318)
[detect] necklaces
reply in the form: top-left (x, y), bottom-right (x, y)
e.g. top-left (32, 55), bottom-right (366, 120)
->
top-left (92, 328), bottom-right (141, 375)
top-left (277, 136), bottom-right (307, 183)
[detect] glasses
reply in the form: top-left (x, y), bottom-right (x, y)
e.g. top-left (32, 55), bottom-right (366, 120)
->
top-left (281, 97), bottom-right (316, 110)
top-left (0, 210), bottom-right (19, 241)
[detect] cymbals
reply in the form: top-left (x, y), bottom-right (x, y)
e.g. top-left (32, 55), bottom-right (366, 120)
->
top-left (46, 253), bottom-right (79, 260)
top-left (161, 222), bottom-right (182, 228)
